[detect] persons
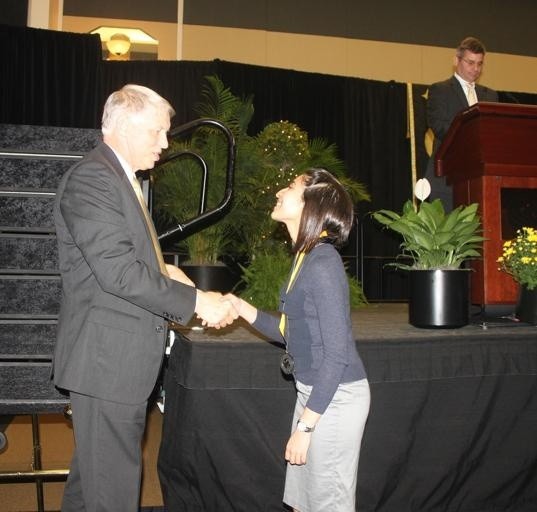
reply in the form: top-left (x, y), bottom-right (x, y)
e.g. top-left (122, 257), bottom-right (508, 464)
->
top-left (50, 80), bottom-right (241, 511)
top-left (196, 167), bottom-right (371, 510)
top-left (420, 36), bottom-right (501, 221)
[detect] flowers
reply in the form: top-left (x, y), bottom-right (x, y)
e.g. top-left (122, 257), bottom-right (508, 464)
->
top-left (362, 177), bottom-right (492, 273)
top-left (495, 225), bottom-right (537, 291)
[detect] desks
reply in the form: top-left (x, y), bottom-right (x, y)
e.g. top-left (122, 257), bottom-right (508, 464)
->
top-left (163, 304), bottom-right (537, 512)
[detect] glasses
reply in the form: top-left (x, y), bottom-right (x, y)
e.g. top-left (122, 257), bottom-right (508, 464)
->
top-left (457, 56), bottom-right (483, 66)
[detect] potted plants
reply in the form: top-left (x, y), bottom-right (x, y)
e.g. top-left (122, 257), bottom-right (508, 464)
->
top-left (146, 69), bottom-right (372, 295)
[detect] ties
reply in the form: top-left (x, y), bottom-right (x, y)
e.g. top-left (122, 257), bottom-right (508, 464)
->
top-left (132, 177), bottom-right (169, 279)
top-left (467, 82), bottom-right (476, 107)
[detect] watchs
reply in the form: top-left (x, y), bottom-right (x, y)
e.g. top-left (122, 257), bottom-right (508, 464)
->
top-left (296, 420), bottom-right (315, 433)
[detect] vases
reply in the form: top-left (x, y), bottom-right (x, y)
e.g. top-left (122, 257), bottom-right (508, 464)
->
top-left (410, 268), bottom-right (470, 328)
top-left (516, 285), bottom-right (537, 324)
